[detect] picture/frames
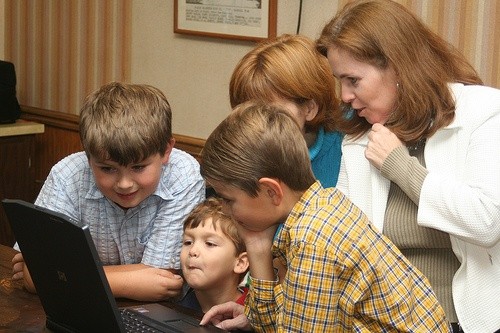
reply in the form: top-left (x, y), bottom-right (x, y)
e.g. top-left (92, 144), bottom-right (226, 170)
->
top-left (174, 0), bottom-right (278, 43)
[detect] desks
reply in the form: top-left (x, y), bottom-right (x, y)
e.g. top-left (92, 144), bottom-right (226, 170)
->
top-left (0, 244), bottom-right (232, 332)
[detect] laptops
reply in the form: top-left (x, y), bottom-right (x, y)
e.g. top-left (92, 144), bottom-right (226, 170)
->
top-left (2, 199), bottom-right (235, 333)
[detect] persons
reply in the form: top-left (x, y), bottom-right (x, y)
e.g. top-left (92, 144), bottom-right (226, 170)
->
top-left (199, 100), bottom-right (454, 333)
top-left (199, 0), bottom-right (500, 333)
top-left (229, 34), bottom-right (355, 245)
top-left (10, 81), bottom-right (207, 302)
top-left (177, 195), bottom-right (249, 314)
